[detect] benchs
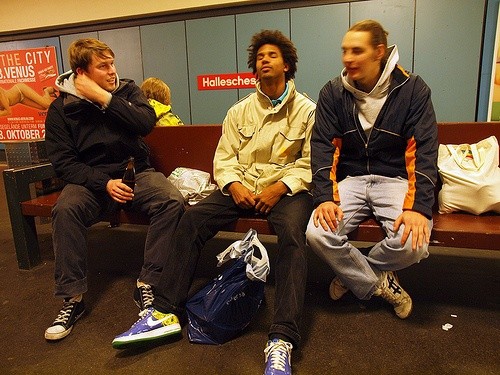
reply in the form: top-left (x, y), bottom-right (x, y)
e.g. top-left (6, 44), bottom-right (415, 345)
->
top-left (2, 122), bottom-right (500, 271)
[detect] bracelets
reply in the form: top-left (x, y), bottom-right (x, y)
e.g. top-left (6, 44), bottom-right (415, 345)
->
top-left (101, 92), bottom-right (113, 110)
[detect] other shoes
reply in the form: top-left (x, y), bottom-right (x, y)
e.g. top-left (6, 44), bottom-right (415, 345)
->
top-left (51, 94), bottom-right (58, 98)
top-left (43, 87), bottom-right (59, 93)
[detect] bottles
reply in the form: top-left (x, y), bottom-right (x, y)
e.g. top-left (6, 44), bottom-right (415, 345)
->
top-left (118, 157), bottom-right (135, 210)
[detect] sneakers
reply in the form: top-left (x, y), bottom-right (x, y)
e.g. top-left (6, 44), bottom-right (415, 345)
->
top-left (112, 307), bottom-right (182, 349)
top-left (263, 337), bottom-right (293, 375)
top-left (45, 293), bottom-right (85, 339)
top-left (131, 277), bottom-right (157, 310)
top-left (329, 275), bottom-right (350, 299)
top-left (369, 271), bottom-right (413, 318)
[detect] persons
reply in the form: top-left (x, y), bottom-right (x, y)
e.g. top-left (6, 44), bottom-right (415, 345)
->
top-left (306, 20), bottom-right (445, 320)
top-left (0, 83), bottom-right (59, 116)
top-left (110, 28), bottom-right (318, 375)
top-left (43, 37), bottom-right (187, 340)
top-left (140, 77), bottom-right (185, 126)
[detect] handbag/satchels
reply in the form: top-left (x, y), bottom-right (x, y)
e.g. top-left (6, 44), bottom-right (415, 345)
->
top-left (216, 227), bottom-right (271, 280)
top-left (168, 167), bottom-right (220, 206)
top-left (437, 135), bottom-right (500, 215)
top-left (185, 260), bottom-right (267, 346)
